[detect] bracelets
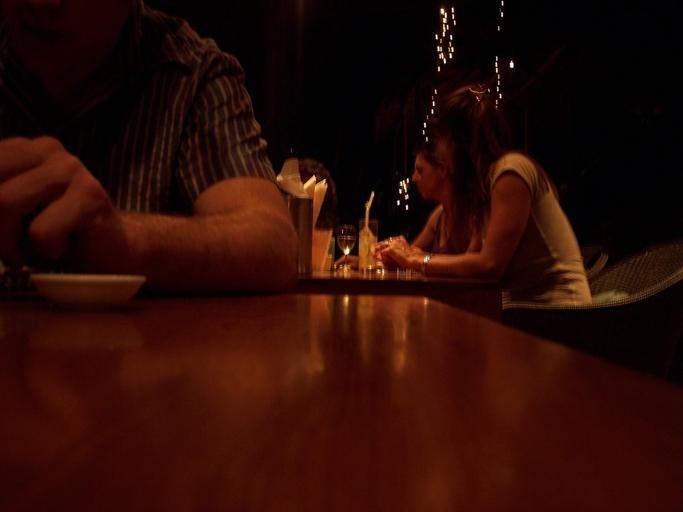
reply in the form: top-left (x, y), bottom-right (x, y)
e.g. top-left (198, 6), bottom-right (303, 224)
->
top-left (423, 253), bottom-right (435, 274)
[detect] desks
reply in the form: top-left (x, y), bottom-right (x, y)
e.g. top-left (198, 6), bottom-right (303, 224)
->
top-left (0, 293), bottom-right (681, 511)
top-left (300, 263), bottom-right (505, 325)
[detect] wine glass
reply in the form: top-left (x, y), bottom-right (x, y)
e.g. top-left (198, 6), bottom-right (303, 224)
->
top-left (336, 225), bottom-right (357, 271)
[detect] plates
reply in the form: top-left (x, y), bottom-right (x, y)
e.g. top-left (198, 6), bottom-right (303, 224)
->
top-left (28, 273), bottom-right (146, 306)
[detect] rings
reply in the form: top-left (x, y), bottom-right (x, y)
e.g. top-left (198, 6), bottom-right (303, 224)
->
top-left (384, 237), bottom-right (392, 245)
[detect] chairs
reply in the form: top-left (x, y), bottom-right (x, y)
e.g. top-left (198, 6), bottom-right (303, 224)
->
top-left (577, 241), bottom-right (615, 278)
top-left (498, 238), bottom-right (683, 385)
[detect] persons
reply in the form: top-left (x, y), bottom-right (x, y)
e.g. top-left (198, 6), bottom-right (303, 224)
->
top-left (0, 0), bottom-right (301, 293)
top-left (333, 145), bottom-right (485, 271)
top-left (379, 83), bottom-right (592, 307)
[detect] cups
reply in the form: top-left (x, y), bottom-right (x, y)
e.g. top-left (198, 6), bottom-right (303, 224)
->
top-left (312, 228), bottom-right (335, 271)
top-left (358, 220), bottom-right (379, 272)
